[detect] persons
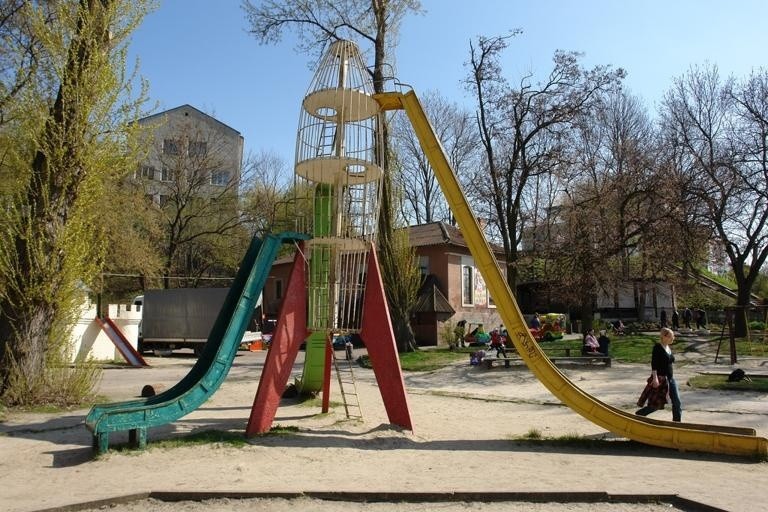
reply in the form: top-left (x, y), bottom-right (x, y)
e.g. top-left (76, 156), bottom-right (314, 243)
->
top-left (635, 325), bottom-right (683, 422)
top-left (489, 322), bottom-right (509, 358)
top-left (659, 307), bottom-right (668, 328)
top-left (531, 311), bottom-right (568, 330)
top-left (694, 305), bottom-right (707, 330)
top-left (597, 328), bottom-right (611, 356)
top-left (584, 327), bottom-right (601, 357)
top-left (682, 306), bottom-right (693, 330)
top-left (582, 328), bottom-right (589, 355)
top-left (455, 319), bottom-right (467, 348)
top-left (670, 309), bottom-right (680, 328)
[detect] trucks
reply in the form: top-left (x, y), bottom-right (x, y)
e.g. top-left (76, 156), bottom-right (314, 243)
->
top-left (126, 285), bottom-right (265, 358)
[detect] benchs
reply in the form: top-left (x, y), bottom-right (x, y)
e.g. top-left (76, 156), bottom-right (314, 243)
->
top-left (541, 348), bottom-right (585, 357)
top-left (482, 356), bottom-right (612, 368)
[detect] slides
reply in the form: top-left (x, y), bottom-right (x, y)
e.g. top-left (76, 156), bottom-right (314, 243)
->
top-left (295, 183), bottom-right (345, 396)
top-left (85, 232), bottom-right (282, 459)
top-left (399, 88), bottom-right (768, 459)
